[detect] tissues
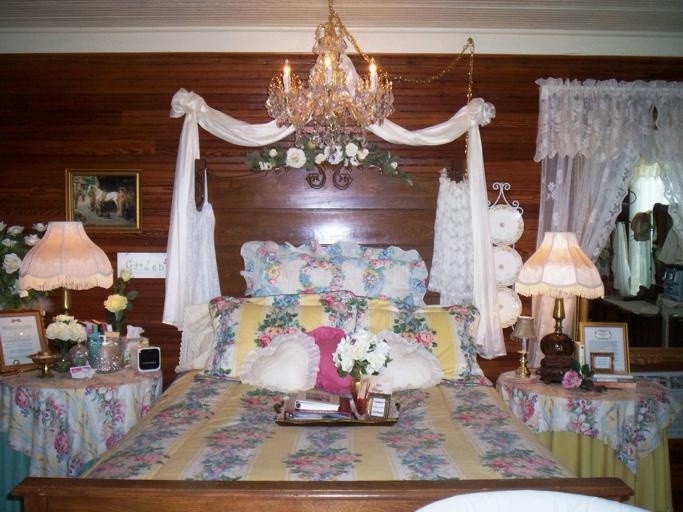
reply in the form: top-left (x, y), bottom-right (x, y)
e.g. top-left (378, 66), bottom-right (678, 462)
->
top-left (124, 324), bottom-right (148, 370)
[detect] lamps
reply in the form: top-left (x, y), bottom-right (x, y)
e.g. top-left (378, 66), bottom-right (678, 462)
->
top-left (511, 316), bottom-right (535, 379)
top-left (265, 0), bottom-right (395, 149)
top-left (515, 231), bottom-right (605, 385)
top-left (18, 221), bottom-right (114, 363)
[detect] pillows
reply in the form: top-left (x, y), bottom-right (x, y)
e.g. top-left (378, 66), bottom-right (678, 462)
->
top-left (361, 331), bottom-right (445, 393)
top-left (239, 331), bottom-right (320, 393)
top-left (196, 290), bottom-right (355, 379)
top-left (354, 295), bottom-right (492, 385)
top-left (239, 239), bottom-right (429, 308)
top-left (307, 327), bottom-right (356, 394)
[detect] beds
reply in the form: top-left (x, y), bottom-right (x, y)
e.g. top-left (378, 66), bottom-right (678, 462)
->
top-left (7, 365), bottom-right (635, 511)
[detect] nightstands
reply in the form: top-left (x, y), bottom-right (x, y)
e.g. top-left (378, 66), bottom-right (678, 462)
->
top-left (496, 371), bottom-right (681, 511)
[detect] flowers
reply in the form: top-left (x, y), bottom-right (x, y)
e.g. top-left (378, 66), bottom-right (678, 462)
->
top-left (0, 221), bottom-right (49, 311)
top-left (560, 361), bottom-right (623, 393)
top-left (45, 314), bottom-right (88, 361)
top-left (104, 270), bottom-right (139, 334)
top-left (331, 330), bottom-right (394, 378)
top-left (244, 134), bottom-right (415, 191)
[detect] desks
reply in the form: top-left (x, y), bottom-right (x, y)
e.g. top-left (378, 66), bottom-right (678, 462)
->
top-left (0, 368), bottom-right (164, 511)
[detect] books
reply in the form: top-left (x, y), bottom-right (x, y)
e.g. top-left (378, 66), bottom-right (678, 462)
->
top-left (78, 319), bottom-right (113, 334)
top-left (587, 374), bottom-right (637, 388)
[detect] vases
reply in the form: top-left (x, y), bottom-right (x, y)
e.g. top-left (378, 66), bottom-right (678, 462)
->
top-left (351, 378), bottom-right (369, 415)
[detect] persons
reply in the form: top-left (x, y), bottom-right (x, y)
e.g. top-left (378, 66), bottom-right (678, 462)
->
top-left (75, 187), bottom-right (136, 222)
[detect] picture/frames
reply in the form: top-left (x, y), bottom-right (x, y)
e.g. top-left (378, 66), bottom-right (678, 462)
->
top-left (0, 308), bottom-right (48, 373)
top-left (577, 321), bottom-right (631, 375)
top-left (64, 168), bottom-right (142, 234)
